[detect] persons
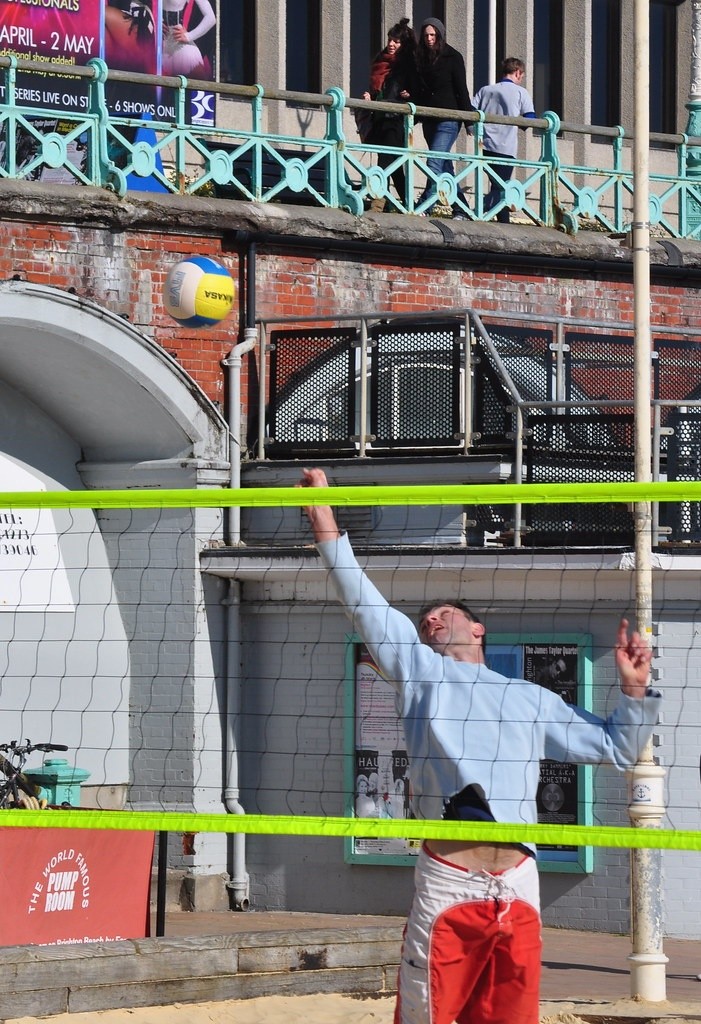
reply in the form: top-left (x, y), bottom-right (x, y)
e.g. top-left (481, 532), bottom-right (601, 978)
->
top-left (361, 18), bottom-right (416, 209)
top-left (356, 772), bottom-right (377, 818)
top-left (389, 779), bottom-right (405, 818)
top-left (297, 468), bottom-right (662, 1024)
top-left (400, 17), bottom-right (476, 219)
top-left (161, 0), bottom-right (216, 76)
top-left (472, 58), bottom-right (535, 220)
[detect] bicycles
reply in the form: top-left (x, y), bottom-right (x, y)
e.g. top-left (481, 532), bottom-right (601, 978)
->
top-left (0, 739), bottom-right (72, 809)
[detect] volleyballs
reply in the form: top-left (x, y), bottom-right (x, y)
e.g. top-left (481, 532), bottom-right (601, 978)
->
top-left (160, 255), bottom-right (238, 332)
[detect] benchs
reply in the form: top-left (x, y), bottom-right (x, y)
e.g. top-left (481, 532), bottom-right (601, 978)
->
top-left (196, 136), bottom-right (391, 214)
